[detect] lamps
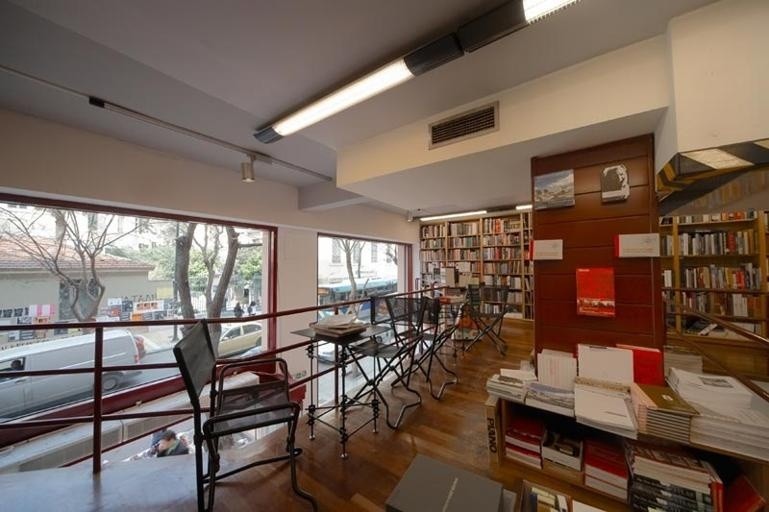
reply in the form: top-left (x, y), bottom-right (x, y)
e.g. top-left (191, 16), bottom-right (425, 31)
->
top-left (253, 0), bottom-right (582, 144)
top-left (240, 154), bottom-right (256, 182)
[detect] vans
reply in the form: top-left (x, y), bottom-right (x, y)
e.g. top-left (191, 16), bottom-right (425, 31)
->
top-left (0, 328), bottom-right (143, 422)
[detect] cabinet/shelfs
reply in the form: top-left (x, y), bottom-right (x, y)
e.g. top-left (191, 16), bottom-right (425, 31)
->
top-left (483, 394), bottom-right (769, 512)
top-left (419, 209), bottom-right (536, 322)
top-left (658, 210), bottom-right (769, 382)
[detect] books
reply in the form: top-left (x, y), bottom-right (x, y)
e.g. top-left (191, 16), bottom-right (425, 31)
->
top-left (657, 208), bottom-right (768, 340)
top-left (486, 342), bottom-right (768, 512)
top-left (576, 266), bottom-right (616, 318)
top-left (385, 208), bottom-right (534, 322)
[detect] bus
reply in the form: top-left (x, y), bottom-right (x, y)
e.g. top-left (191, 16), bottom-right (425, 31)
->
top-left (318, 277), bottom-right (397, 318)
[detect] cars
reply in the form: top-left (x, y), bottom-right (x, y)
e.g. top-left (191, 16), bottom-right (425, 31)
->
top-left (218, 320), bottom-right (262, 359)
top-left (318, 340), bottom-right (355, 363)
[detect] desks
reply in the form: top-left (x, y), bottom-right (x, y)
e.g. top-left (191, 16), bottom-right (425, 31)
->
top-left (289, 320), bottom-right (391, 458)
top-left (429, 295), bottom-right (468, 358)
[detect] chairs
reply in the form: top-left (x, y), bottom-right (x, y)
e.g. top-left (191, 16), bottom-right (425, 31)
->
top-left (385, 296), bottom-right (458, 399)
top-left (174, 319), bottom-right (316, 512)
top-left (343, 294), bottom-right (428, 431)
top-left (460, 284), bottom-right (512, 355)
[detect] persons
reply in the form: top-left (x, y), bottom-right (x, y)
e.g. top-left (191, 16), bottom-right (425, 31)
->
top-left (248, 301), bottom-right (257, 317)
top-left (147, 427), bottom-right (168, 457)
top-left (3, 360), bottom-right (23, 380)
top-left (234, 302), bottom-right (246, 317)
top-left (157, 431), bottom-right (189, 455)
